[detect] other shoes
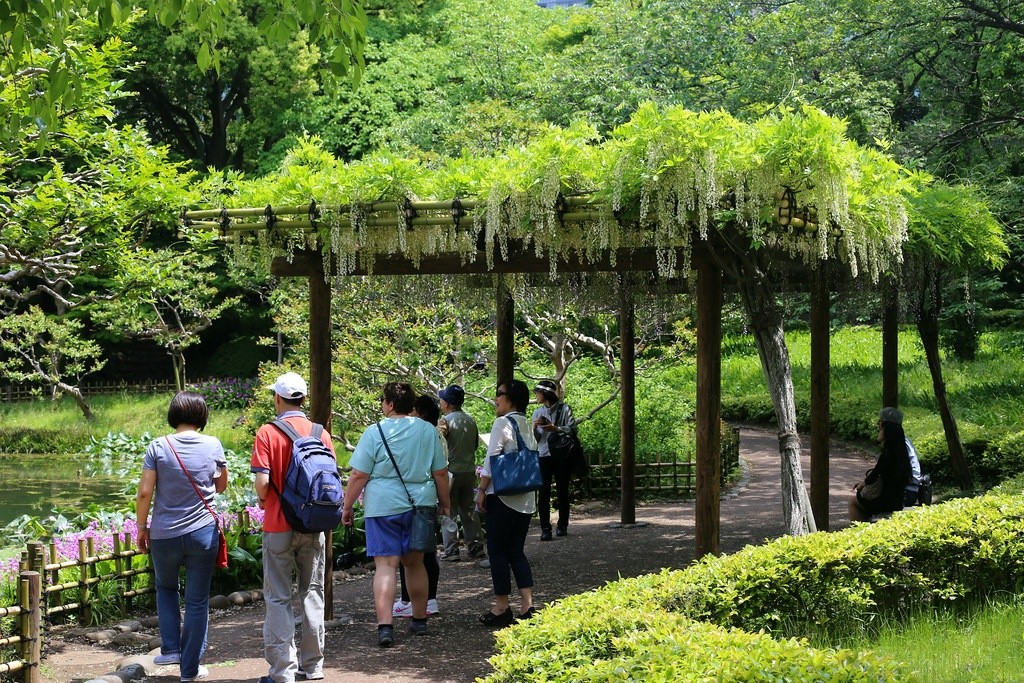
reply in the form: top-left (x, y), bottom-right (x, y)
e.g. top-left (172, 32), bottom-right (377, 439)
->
top-left (258, 675), bottom-right (276, 683)
top-left (440, 548), bottom-right (461, 562)
top-left (409, 620), bottom-right (429, 635)
top-left (295, 664), bottom-right (324, 680)
top-left (540, 529), bottom-right (553, 541)
top-left (466, 543), bottom-right (486, 557)
top-left (555, 525), bottom-right (567, 536)
top-left (377, 626), bottom-right (395, 645)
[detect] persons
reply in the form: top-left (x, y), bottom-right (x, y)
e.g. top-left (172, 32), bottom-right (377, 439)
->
top-left (136, 391), bottom-right (227, 682)
top-left (390, 380), bottom-right (583, 627)
top-left (850, 407), bottom-right (921, 525)
top-left (250, 371), bottom-right (337, 683)
top-left (342, 382), bottom-right (449, 649)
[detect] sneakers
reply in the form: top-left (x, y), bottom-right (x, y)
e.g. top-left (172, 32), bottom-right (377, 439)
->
top-left (154, 653), bottom-right (181, 665)
top-left (426, 599), bottom-right (439, 615)
top-left (392, 597), bottom-right (413, 617)
top-left (180, 664), bottom-right (209, 681)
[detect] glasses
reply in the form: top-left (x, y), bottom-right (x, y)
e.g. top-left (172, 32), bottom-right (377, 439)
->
top-left (380, 395), bottom-right (386, 403)
top-left (496, 390), bottom-right (508, 397)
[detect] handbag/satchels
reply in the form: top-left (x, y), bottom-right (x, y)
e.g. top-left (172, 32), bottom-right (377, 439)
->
top-left (409, 510), bottom-right (438, 554)
top-left (213, 530), bottom-right (228, 567)
top-left (917, 473), bottom-right (933, 506)
top-left (489, 416), bottom-right (544, 497)
top-left (547, 402), bottom-right (581, 460)
top-left (860, 468), bottom-right (884, 514)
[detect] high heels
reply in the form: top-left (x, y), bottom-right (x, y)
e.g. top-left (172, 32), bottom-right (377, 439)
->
top-left (477, 605), bottom-right (513, 626)
top-left (512, 606), bottom-right (535, 626)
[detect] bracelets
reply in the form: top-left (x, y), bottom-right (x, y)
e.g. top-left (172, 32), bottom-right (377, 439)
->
top-left (137, 520), bottom-right (146, 530)
top-left (478, 486), bottom-right (487, 491)
top-left (258, 495), bottom-right (265, 501)
top-left (558, 426), bottom-right (561, 431)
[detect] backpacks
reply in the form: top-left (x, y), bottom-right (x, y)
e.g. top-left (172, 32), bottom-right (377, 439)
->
top-left (264, 420), bottom-right (343, 534)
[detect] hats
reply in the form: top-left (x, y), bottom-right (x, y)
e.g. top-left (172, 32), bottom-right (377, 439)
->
top-left (438, 384), bottom-right (465, 405)
top-left (879, 405), bottom-right (905, 425)
top-left (534, 380), bottom-right (559, 401)
top-left (265, 372), bottom-right (307, 400)
